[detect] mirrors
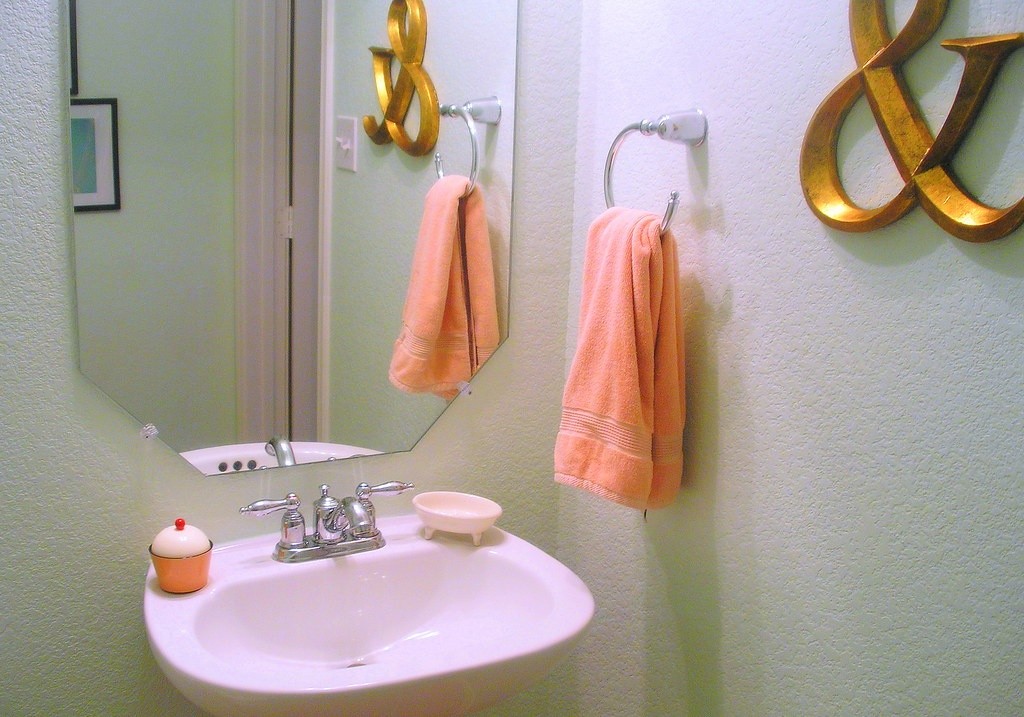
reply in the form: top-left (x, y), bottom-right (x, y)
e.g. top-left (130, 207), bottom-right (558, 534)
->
top-left (58, 0), bottom-right (519, 477)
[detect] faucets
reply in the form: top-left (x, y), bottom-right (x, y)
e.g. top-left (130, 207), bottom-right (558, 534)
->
top-left (265, 434), bottom-right (298, 467)
top-left (239, 483), bottom-right (414, 561)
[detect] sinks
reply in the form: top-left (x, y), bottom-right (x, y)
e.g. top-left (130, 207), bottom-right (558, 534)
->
top-left (178, 442), bottom-right (388, 476)
top-left (144, 513), bottom-right (596, 716)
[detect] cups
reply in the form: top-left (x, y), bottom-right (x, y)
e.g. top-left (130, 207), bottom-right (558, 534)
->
top-left (148, 539), bottom-right (213, 593)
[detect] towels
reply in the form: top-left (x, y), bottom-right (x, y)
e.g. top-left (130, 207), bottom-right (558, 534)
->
top-left (554, 208), bottom-right (685, 507)
top-left (391, 175), bottom-right (500, 404)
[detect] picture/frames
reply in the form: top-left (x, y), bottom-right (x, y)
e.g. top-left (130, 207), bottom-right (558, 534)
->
top-left (66, 0), bottom-right (79, 96)
top-left (70, 98), bottom-right (121, 212)
